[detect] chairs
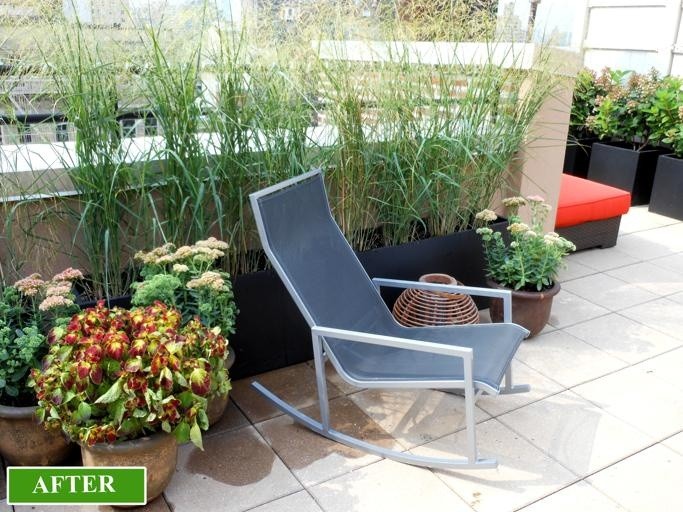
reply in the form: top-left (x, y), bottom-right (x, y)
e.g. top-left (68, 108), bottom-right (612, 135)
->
top-left (248, 169), bottom-right (532, 472)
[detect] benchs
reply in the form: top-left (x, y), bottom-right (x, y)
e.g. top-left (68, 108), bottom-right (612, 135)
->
top-left (555, 172), bottom-right (631, 252)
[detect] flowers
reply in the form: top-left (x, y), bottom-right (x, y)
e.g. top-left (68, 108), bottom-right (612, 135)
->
top-left (1, 266), bottom-right (83, 406)
top-left (474, 195), bottom-right (577, 290)
top-left (644, 71), bottom-right (683, 158)
top-left (127, 234), bottom-right (238, 345)
top-left (587, 65), bottom-right (655, 151)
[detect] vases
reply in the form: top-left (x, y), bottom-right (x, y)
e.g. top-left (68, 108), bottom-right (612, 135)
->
top-left (0, 403), bottom-right (70, 467)
top-left (648, 150), bottom-right (683, 222)
top-left (587, 137), bottom-right (658, 208)
top-left (486, 277), bottom-right (559, 341)
top-left (201, 342), bottom-right (235, 425)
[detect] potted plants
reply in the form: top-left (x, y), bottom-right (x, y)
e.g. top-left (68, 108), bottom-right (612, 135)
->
top-left (566, 66), bottom-right (596, 181)
top-left (29, 297), bottom-right (233, 506)
